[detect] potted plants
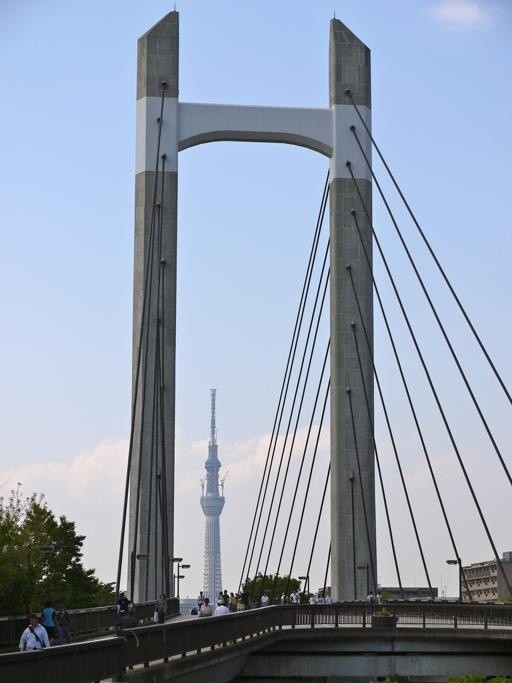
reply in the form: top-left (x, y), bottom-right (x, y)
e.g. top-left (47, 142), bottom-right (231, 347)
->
top-left (371, 607), bottom-right (399, 633)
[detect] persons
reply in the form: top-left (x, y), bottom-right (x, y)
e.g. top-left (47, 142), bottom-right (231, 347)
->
top-left (196, 588), bottom-right (332, 619)
top-left (41, 599), bottom-right (65, 646)
top-left (376, 591), bottom-right (383, 604)
top-left (366, 591), bottom-right (377, 604)
top-left (190, 606), bottom-right (198, 615)
top-left (18, 614), bottom-right (50, 652)
top-left (123, 607), bottom-right (138, 628)
top-left (116, 592), bottom-right (133, 616)
top-left (154, 594), bottom-right (167, 624)
top-left (56, 604), bottom-right (74, 645)
